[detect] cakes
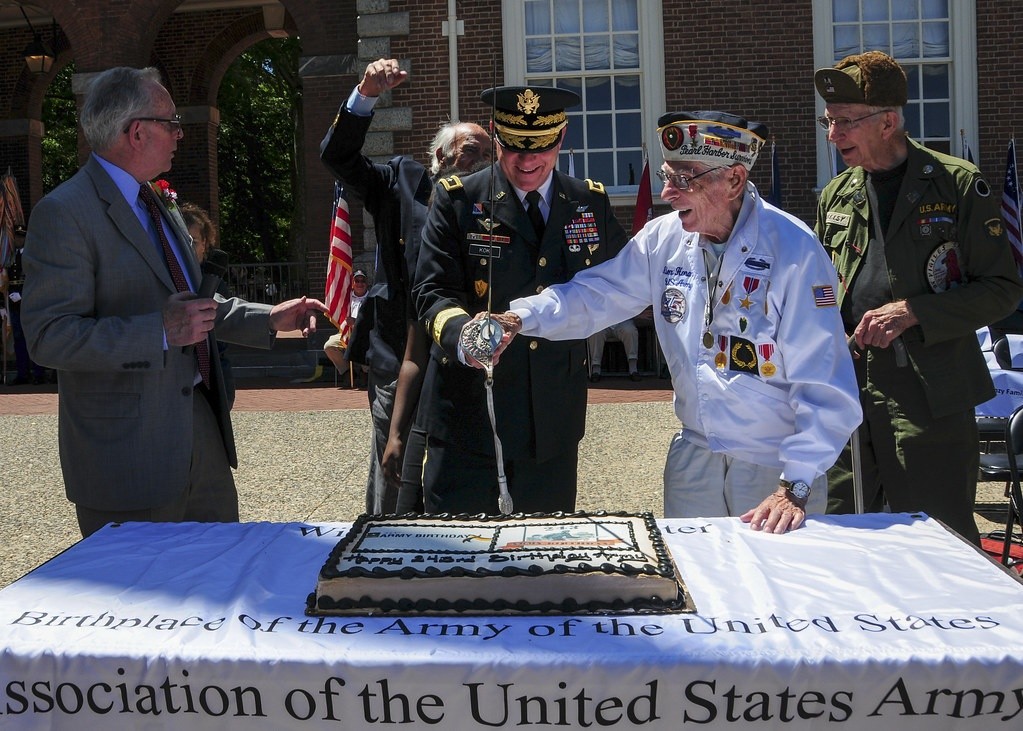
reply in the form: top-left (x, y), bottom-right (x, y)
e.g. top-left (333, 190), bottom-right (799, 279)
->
top-left (305, 510), bottom-right (686, 613)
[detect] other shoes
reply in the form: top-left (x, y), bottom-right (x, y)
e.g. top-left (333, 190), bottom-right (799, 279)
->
top-left (629, 371), bottom-right (643, 381)
top-left (9, 378), bottom-right (33, 386)
top-left (34, 380), bottom-right (46, 385)
top-left (590, 370), bottom-right (601, 383)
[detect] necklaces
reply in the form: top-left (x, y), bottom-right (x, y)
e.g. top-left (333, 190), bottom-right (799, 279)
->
top-left (703, 250), bottom-right (725, 349)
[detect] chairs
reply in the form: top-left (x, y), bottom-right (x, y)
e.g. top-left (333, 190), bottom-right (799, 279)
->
top-left (973, 407), bottom-right (1023, 572)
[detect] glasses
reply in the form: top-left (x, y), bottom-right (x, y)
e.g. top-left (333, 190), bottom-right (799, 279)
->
top-left (123, 115), bottom-right (181, 132)
top-left (655, 165), bottom-right (735, 189)
top-left (816, 109), bottom-right (895, 131)
top-left (353, 279), bottom-right (366, 283)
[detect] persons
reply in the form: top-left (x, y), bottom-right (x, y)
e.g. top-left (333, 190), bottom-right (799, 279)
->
top-left (589, 309), bottom-right (653, 383)
top-left (473, 108), bottom-right (863, 534)
top-left (182, 203), bottom-right (235, 410)
top-left (324, 270), bottom-right (371, 387)
top-left (319, 58), bottom-right (497, 514)
top-left (20, 67), bottom-right (331, 534)
top-left (814, 50), bottom-right (1023, 553)
top-left (0, 224), bottom-right (48, 386)
top-left (411, 85), bottom-right (630, 515)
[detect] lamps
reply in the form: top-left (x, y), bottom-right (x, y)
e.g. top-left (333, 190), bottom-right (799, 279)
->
top-left (15, 4), bottom-right (56, 74)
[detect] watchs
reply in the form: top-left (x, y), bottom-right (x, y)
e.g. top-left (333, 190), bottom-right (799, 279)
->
top-left (778, 480), bottom-right (811, 498)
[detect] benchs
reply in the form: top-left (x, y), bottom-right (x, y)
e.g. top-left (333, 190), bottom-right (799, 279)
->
top-left (307, 329), bottom-right (339, 387)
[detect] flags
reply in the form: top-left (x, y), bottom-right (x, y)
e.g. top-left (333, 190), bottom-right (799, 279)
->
top-left (811, 285), bottom-right (837, 308)
top-left (321, 184), bottom-right (352, 347)
top-left (0, 174), bottom-right (25, 267)
top-left (632, 160), bottom-right (653, 321)
top-left (1000, 136), bottom-right (1023, 269)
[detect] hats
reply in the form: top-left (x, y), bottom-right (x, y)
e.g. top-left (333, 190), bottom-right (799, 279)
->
top-left (352, 269), bottom-right (367, 278)
top-left (656, 110), bottom-right (769, 172)
top-left (14, 225), bottom-right (27, 237)
top-left (814, 51), bottom-right (907, 107)
top-left (480, 85), bottom-right (581, 154)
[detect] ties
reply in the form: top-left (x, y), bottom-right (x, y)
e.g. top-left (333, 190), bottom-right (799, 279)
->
top-left (524, 190), bottom-right (546, 245)
top-left (138, 185), bottom-right (209, 388)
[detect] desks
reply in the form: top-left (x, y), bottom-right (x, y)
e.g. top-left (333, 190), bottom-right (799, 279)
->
top-left (0, 512), bottom-right (1023, 731)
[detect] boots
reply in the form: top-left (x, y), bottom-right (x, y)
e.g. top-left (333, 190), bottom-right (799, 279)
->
top-left (341, 367), bottom-right (359, 390)
top-left (358, 367), bottom-right (368, 390)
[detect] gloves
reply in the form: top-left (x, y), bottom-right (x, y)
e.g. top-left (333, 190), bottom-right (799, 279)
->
top-left (9, 292), bottom-right (21, 302)
top-left (0, 308), bottom-right (7, 321)
